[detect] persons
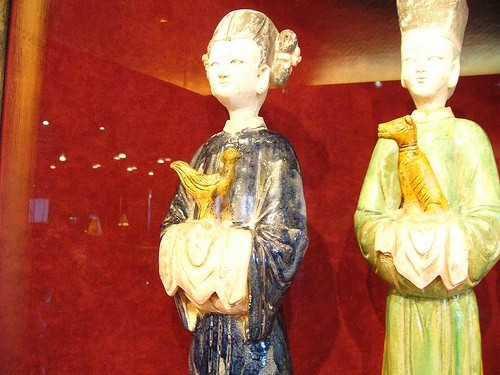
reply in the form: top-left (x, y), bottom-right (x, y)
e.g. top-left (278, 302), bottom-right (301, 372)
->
top-left (351, 0), bottom-right (500, 375)
top-left (157, 9), bottom-right (311, 375)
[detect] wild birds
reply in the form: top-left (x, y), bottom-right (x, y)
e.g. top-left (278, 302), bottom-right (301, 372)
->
top-left (169, 147), bottom-right (243, 220)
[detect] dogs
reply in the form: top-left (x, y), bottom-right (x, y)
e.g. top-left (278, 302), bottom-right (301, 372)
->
top-left (377, 114), bottom-right (451, 213)
